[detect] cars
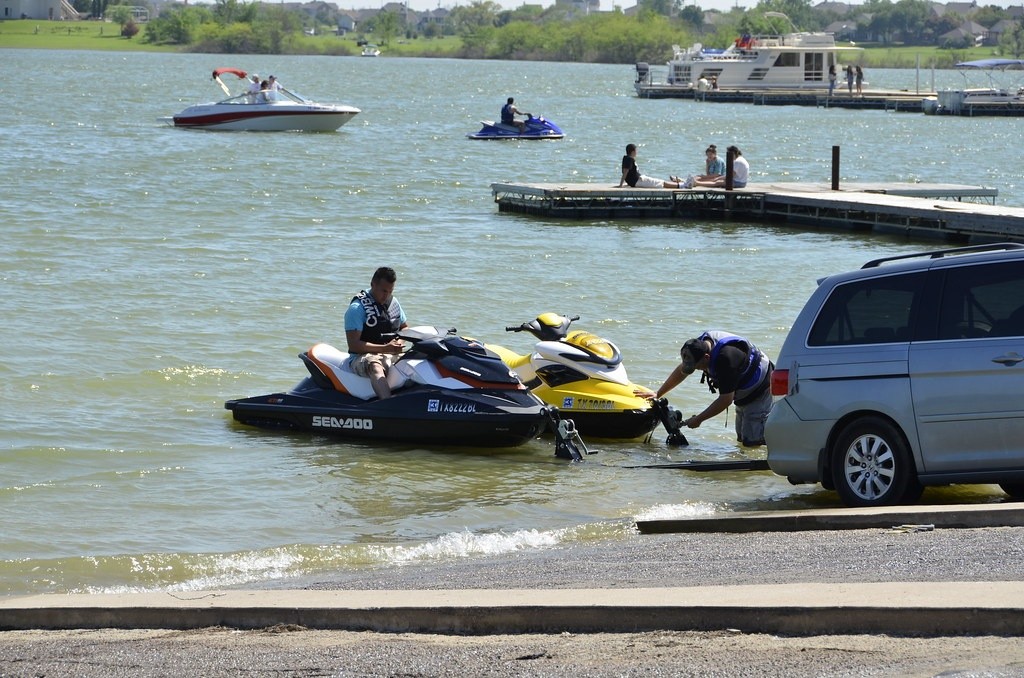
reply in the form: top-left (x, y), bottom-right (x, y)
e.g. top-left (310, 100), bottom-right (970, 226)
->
top-left (304, 27), bottom-right (314, 36)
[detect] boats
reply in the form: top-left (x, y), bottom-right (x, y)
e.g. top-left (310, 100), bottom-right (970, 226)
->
top-left (920, 58), bottom-right (1023, 115)
top-left (361, 44), bottom-right (381, 58)
top-left (634, 11), bottom-right (870, 99)
top-left (154, 69), bottom-right (362, 134)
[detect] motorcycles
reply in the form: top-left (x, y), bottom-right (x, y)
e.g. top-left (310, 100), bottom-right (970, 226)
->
top-left (459, 310), bottom-right (664, 440)
top-left (468, 112), bottom-right (566, 139)
top-left (225, 324), bottom-right (548, 447)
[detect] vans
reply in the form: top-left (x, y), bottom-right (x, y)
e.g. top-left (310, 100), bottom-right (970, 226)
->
top-left (762, 239), bottom-right (1023, 508)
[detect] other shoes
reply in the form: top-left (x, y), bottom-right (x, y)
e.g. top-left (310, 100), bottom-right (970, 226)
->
top-left (670, 175), bottom-right (676, 182)
top-left (685, 171), bottom-right (696, 189)
top-left (676, 176), bottom-right (680, 183)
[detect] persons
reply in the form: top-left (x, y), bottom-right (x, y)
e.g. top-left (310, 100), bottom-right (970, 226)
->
top-left (846, 65), bottom-right (863, 95)
top-left (633, 330), bottom-right (775, 447)
top-left (743, 32), bottom-right (753, 47)
top-left (613, 144), bottom-right (693, 189)
top-left (669, 145), bottom-right (749, 188)
top-left (345, 266), bottom-right (408, 400)
top-left (501, 98), bottom-right (531, 133)
top-left (248, 74), bottom-right (283, 103)
top-left (828, 65), bottom-right (836, 96)
top-left (697, 74), bottom-right (718, 91)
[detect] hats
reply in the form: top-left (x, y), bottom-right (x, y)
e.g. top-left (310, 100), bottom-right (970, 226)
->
top-left (680, 339), bottom-right (707, 374)
